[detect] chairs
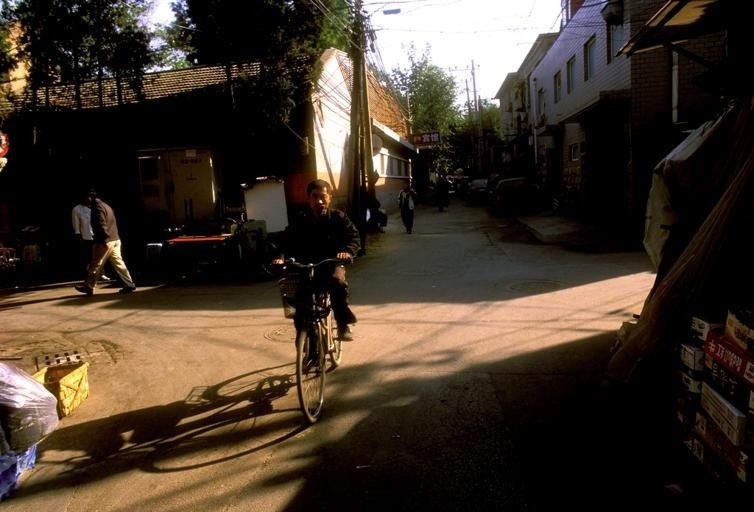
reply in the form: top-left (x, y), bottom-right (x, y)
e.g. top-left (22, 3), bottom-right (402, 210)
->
top-left (118, 283), bottom-right (136, 293)
top-left (75, 284), bottom-right (93, 295)
top-left (337, 324), bottom-right (354, 342)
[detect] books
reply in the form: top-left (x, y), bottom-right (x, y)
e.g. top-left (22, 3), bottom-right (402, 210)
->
top-left (32, 362), bottom-right (90, 419)
top-left (677, 307), bottom-right (754, 512)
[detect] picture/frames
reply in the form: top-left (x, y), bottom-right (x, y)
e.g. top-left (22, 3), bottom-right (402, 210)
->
top-left (166, 232), bottom-right (235, 288)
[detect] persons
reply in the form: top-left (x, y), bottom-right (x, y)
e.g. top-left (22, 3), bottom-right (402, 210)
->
top-left (364, 187), bottom-right (388, 234)
top-left (426, 171), bottom-right (460, 214)
top-left (267, 179), bottom-right (363, 372)
top-left (398, 181), bottom-right (418, 234)
top-left (70, 193), bottom-right (112, 281)
top-left (75, 188), bottom-right (137, 294)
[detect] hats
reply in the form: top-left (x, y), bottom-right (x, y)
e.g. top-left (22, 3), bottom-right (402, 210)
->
top-left (453, 176), bottom-right (541, 211)
